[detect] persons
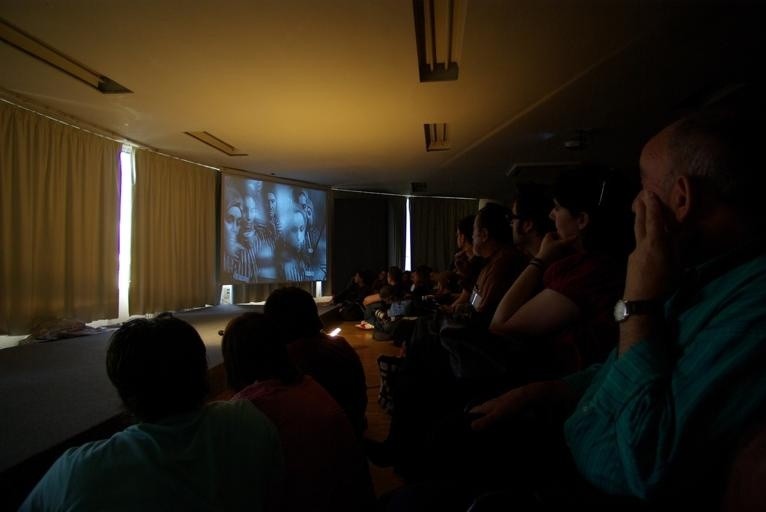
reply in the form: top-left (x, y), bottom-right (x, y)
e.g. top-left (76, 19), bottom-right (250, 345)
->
top-left (224, 191), bottom-right (320, 281)
top-left (433, 114), bottom-right (765, 510)
top-left (19, 312), bottom-right (285, 510)
top-left (379, 165), bottom-right (625, 510)
top-left (221, 314), bottom-right (351, 511)
top-left (265, 291), bottom-right (369, 423)
top-left (336, 266), bottom-right (402, 330)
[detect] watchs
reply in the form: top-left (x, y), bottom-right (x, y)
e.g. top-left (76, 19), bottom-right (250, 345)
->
top-left (613, 296), bottom-right (661, 323)
